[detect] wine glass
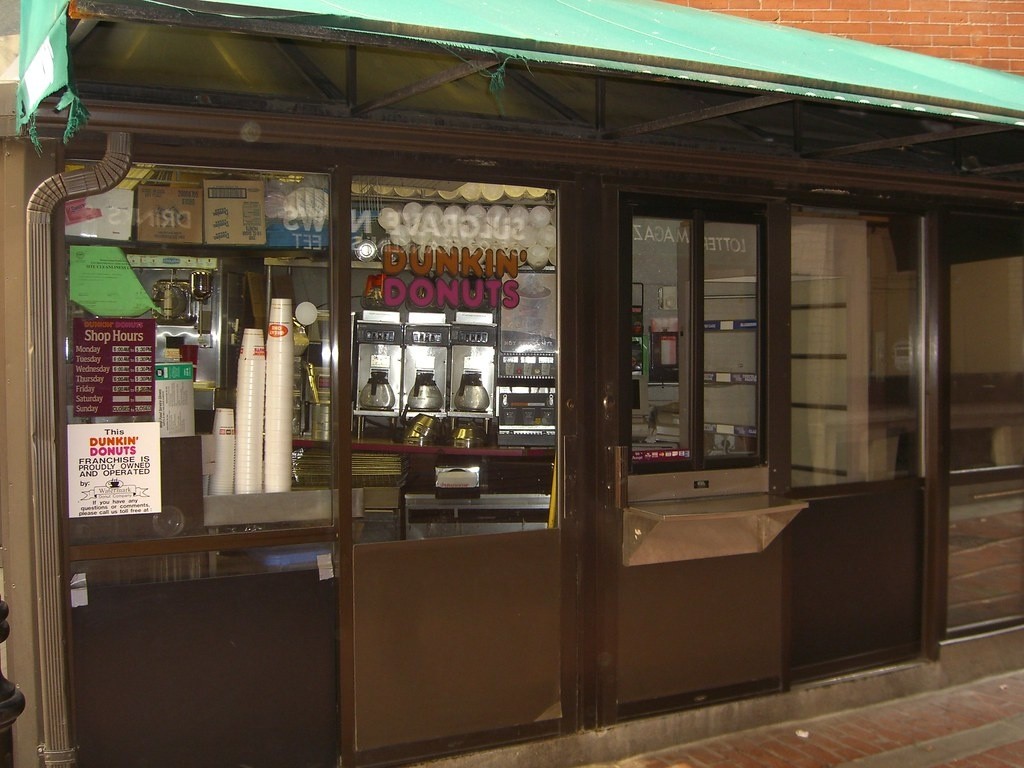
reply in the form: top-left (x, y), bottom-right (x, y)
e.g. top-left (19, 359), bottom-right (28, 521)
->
top-left (191, 270), bottom-right (211, 348)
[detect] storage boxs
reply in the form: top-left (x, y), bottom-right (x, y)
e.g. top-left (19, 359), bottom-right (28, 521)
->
top-left (203, 180), bottom-right (267, 246)
top-left (139, 184), bottom-right (204, 245)
top-left (66, 190), bottom-right (135, 239)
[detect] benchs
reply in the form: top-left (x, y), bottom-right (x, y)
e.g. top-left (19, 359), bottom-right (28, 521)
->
top-left (842, 402), bottom-right (1024, 477)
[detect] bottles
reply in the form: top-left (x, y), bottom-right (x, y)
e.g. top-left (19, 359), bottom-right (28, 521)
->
top-left (292, 389), bottom-right (301, 436)
top-left (311, 375), bottom-right (332, 442)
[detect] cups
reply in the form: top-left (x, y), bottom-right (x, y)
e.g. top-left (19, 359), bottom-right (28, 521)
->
top-left (506, 362), bottom-right (514, 375)
top-left (180, 345), bottom-right (198, 382)
top-left (352, 177), bottom-right (557, 269)
top-left (519, 287), bottom-right (551, 307)
top-left (208, 298), bottom-right (291, 495)
top-left (153, 280), bottom-right (199, 326)
top-left (541, 364), bottom-right (550, 376)
top-left (524, 362), bottom-right (532, 376)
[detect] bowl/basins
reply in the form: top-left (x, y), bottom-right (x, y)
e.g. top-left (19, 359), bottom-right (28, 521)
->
top-left (402, 413), bottom-right (487, 448)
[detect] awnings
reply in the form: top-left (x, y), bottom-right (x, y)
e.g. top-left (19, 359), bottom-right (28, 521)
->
top-left (18, 2), bottom-right (1024, 153)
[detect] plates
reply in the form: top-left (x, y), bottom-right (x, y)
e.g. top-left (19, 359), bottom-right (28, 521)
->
top-left (378, 238), bottom-right (398, 262)
top-left (355, 239), bottom-right (378, 263)
top-left (295, 302), bottom-right (318, 326)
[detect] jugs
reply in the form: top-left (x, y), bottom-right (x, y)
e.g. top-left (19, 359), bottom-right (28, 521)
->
top-left (359, 369), bottom-right (395, 411)
top-left (408, 370), bottom-right (443, 412)
top-left (455, 370), bottom-right (489, 412)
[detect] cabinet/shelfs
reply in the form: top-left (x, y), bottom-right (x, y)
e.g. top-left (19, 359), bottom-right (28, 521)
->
top-left (55, 141), bottom-right (340, 587)
top-left (264, 256), bottom-right (501, 443)
